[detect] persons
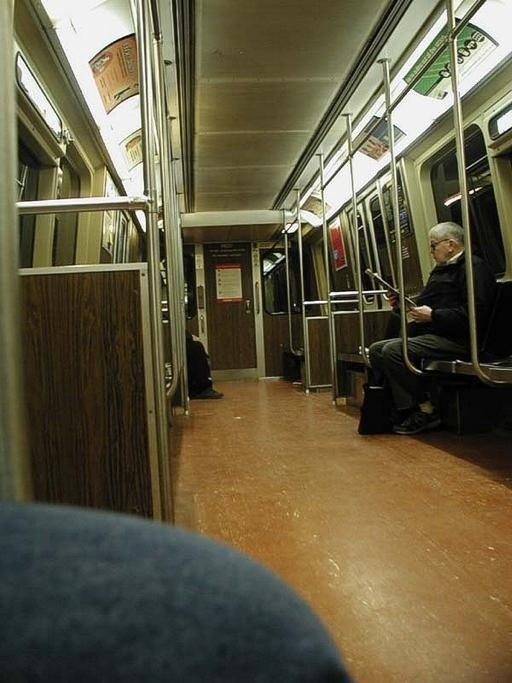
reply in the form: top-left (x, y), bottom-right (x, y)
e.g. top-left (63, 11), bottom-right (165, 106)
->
top-left (368, 220), bottom-right (496, 436)
top-left (185, 329), bottom-right (224, 399)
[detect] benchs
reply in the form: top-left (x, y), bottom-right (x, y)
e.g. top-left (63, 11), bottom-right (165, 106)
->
top-left (329, 308), bottom-right (512, 440)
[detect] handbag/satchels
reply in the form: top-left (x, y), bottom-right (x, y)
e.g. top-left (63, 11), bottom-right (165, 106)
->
top-left (356, 380), bottom-right (394, 435)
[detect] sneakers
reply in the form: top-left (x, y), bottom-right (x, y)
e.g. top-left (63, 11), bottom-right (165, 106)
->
top-left (189, 387), bottom-right (224, 400)
top-left (392, 407), bottom-right (449, 435)
